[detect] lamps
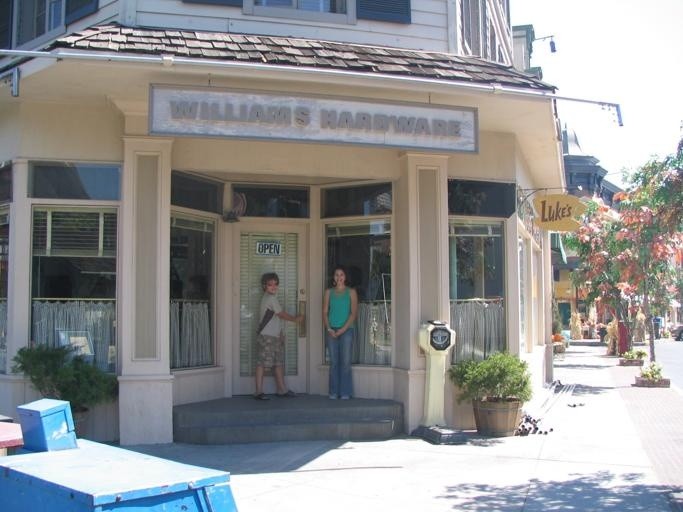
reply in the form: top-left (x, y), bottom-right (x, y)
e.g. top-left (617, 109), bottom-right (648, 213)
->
top-left (222, 211), bottom-right (240, 224)
top-left (534, 35), bottom-right (556, 53)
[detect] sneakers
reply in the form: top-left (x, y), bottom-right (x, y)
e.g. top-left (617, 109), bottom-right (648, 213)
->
top-left (329, 394), bottom-right (337, 400)
top-left (341, 393), bottom-right (351, 400)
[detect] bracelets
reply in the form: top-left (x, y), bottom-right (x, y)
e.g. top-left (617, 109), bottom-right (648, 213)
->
top-left (327, 327), bottom-right (330, 332)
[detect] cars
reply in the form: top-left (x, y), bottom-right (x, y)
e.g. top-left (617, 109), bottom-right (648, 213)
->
top-left (669, 324), bottom-right (683, 342)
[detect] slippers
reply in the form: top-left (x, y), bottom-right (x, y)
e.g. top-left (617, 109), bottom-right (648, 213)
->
top-left (254, 392), bottom-right (270, 400)
top-left (275, 390), bottom-right (295, 397)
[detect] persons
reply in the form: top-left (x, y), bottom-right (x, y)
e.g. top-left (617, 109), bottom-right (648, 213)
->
top-left (253, 271), bottom-right (307, 402)
top-left (322, 265), bottom-right (360, 403)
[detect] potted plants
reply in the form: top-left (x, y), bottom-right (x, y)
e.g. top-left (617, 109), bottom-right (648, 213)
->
top-left (8, 343), bottom-right (117, 439)
top-left (445, 350), bottom-right (533, 438)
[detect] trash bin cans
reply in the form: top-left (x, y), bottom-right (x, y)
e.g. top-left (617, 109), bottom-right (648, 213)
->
top-left (653, 317), bottom-right (662, 340)
